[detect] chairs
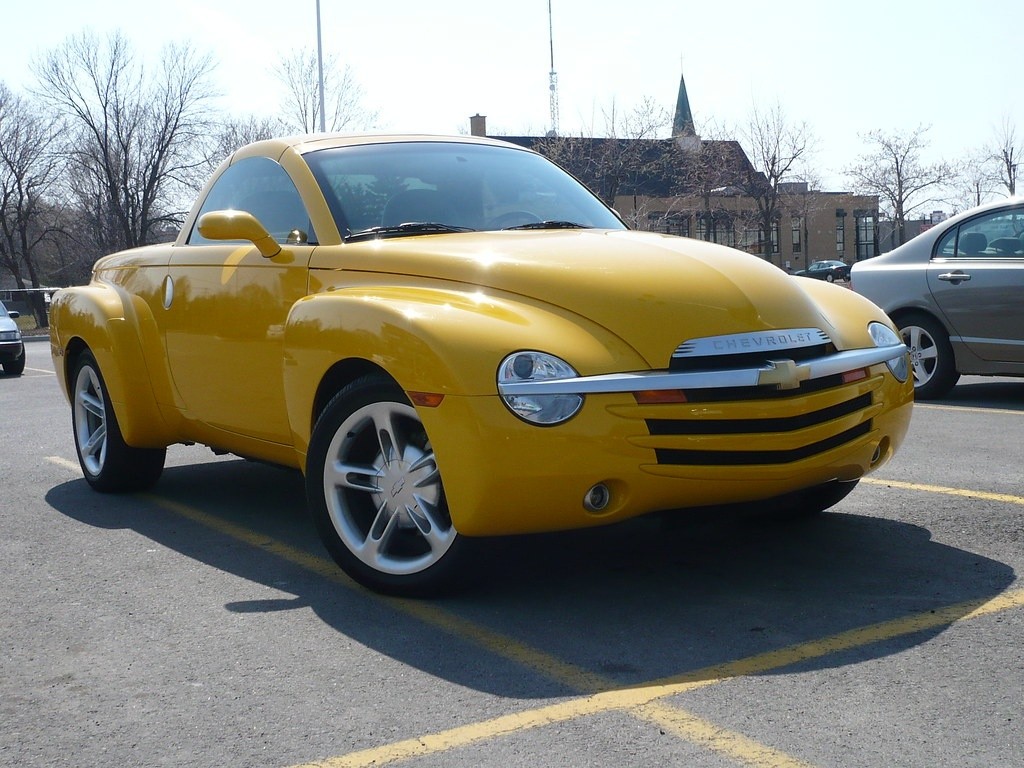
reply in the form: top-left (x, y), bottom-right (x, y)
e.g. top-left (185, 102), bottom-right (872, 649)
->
top-left (381, 188), bottom-right (468, 228)
top-left (239, 191), bottom-right (306, 235)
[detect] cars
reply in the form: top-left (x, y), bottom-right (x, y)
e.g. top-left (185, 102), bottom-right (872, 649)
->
top-left (850, 195), bottom-right (1024, 403)
top-left (794, 260), bottom-right (851, 283)
top-left (0, 300), bottom-right (25, 375)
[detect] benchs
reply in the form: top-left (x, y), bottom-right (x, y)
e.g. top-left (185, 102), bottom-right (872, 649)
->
top-left (957, 233), bottom-right (1024, 258)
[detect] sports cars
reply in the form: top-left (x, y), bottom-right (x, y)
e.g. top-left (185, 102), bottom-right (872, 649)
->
top-left (49, 131), bottom-right (914, 597)
